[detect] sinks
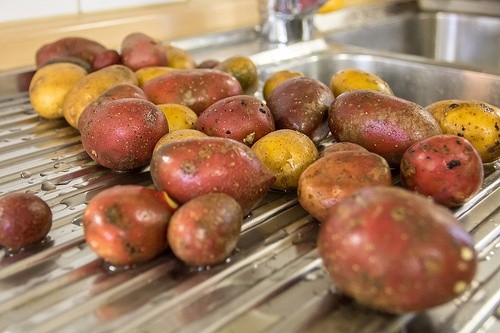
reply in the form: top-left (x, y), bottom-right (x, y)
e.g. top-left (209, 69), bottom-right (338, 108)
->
top-left (254, 49), bottom-right (500, 105)
top-left (328, 11), bottom-right (500, 76)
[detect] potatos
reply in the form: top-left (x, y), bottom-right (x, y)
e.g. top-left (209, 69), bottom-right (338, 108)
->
top-left (0, 192), bottom-right (52, 249)
top-left (317, 184), bottom-right (477, 313)
top-left (28, 32), bottom-right (500, 265)
